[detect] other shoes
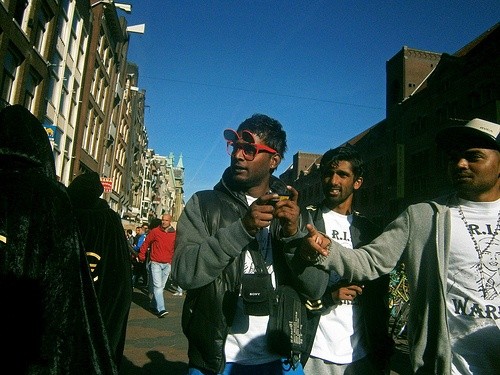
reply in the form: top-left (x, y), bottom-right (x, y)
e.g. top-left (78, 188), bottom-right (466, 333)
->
top-left (158, 310), bottom-right (168, 317)
top-left (173, 292), bottom-right (183, 296)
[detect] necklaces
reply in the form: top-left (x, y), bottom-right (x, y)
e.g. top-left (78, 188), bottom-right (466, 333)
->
top-left (458, 205), bottom-right (499, 299)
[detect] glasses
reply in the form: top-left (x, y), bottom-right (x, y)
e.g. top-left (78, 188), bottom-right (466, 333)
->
top-left (223, 129), bottom-right (277, 161)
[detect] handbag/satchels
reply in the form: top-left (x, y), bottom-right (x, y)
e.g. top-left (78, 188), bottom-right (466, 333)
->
top-left (242, 274), bottom-right (273, 316)
top-left (265, 284), bottom-right (308, 370)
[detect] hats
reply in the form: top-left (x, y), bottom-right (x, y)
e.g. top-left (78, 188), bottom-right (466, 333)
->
top-left (436, 118), bottom-right (500, 147)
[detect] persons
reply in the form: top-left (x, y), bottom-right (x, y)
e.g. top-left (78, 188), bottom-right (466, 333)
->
top-left (137, 213), bottom-right (176, 318)
top-left (304, 147), bottom-right (390, 375)
top-left (68, 172), bottom-right (134, 374)
top-left (127, 218), bottom-right (189, 299)
top-left (300, 117), bottom-right (500, 375)
top-left (171, 113), bottom-right (330, 375)
top-left (1, 104), bottom-right (118, 374)
